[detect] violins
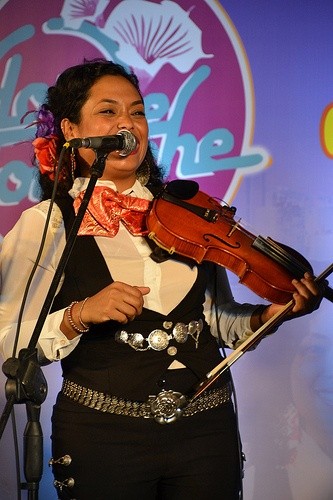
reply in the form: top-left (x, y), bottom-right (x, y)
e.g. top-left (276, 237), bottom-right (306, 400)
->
top-left (145, 179), bottom-right (333, 305)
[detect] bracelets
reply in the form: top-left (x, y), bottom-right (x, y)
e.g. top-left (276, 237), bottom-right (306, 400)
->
top-left (78, 297), bottom-right (91, 329)
top-left (67, 301), bottom-right (90, 333)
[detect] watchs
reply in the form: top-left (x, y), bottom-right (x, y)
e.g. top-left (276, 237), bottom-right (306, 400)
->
top-left (258, 303), bottom-right (284, 334)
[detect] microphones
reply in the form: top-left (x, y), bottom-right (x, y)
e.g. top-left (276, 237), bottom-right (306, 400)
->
top-left (68, 130), bottom-right (136, 157)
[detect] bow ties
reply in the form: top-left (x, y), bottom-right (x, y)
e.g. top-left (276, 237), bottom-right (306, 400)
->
top-left (73, 187), bottom-right (154, 236)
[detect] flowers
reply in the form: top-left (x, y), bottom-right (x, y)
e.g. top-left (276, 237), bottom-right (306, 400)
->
top-left (21, 107), bottom-right (69, 182)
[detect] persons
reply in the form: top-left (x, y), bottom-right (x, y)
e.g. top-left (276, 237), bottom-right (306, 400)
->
top-left (0, 55), bottom-right (329, 500)
